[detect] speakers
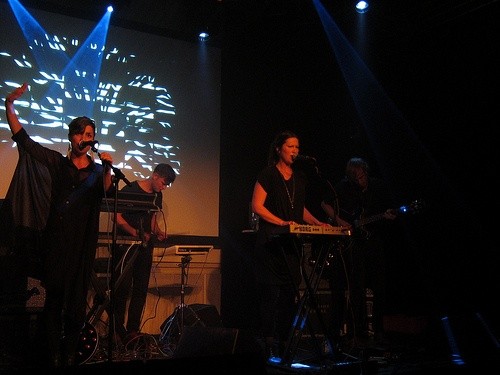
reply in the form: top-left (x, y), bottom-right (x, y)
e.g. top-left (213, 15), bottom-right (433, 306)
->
top-left (273, 291), bottom-right (334, 339)
top-left (173, 327), bottom-right (266, 375)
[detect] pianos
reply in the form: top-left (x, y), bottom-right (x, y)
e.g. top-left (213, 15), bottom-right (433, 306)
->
top-left (269, 224), bottom-right (354, 368)
top-left (92, 189), bottom-right (225, 360)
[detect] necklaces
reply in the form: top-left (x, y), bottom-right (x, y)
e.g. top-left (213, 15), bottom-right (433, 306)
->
top-left (282, 174), bottom-right (296, 209)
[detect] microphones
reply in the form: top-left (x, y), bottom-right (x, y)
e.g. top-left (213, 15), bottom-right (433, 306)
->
top-left (78, 139), bottom-right (98, 148)
top-left (291, 154), bottom-right (316, 160)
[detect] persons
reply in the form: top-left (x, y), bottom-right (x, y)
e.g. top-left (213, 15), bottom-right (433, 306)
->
top-left (321, 157), bottom-right (397, 343)
top-left (111, 163), bottom-right (175, 347)
top-left (252, 131), bottom-right (332, 366)
top-left (4, 82), bottom-right (113, 353)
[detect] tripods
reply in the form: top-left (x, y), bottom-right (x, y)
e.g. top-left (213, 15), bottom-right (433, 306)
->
top-left (158, 263), bottom-right (205, 343)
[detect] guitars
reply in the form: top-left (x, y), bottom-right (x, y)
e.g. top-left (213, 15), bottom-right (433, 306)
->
top-left (332, 200), bottom-right (426, 252)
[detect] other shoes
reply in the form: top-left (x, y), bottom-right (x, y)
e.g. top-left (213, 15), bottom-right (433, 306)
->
top-left (263, 342), bottom-right (282, 366)
